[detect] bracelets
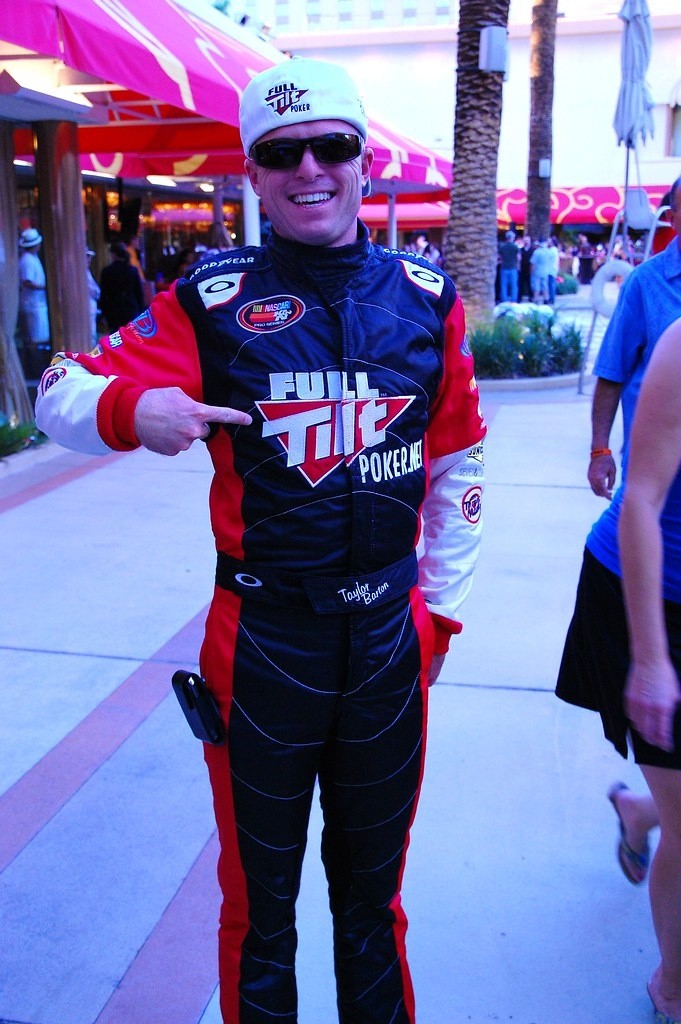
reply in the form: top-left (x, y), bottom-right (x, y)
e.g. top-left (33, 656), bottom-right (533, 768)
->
top-left (591, 449), bottom-right (612, 458)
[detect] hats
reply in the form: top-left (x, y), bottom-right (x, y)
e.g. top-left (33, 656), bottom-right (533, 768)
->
top-left (19, 229), bottom-right (43, 248)
top-left (85, 247), bottom-right (96, 257)
top-left (238, 56), bottom-right (371, 197)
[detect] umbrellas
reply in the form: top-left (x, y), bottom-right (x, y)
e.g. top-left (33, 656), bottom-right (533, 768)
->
top-left (613, 1), bottom-right (655, 211)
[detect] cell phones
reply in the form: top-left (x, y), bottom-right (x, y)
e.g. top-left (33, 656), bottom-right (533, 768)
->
top-left (184, 678), bottom-right (225, 743)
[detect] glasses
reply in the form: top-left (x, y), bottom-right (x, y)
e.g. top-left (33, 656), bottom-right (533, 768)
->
top-left (249, 132), bottom-right (364, 170)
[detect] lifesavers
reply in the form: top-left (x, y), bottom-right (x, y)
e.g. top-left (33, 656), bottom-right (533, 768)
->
top-left (589, 259), bottom-right (635, 319)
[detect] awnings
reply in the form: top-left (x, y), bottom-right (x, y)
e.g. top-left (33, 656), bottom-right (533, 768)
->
top-left (0, 0), bottom-right (455, 251)
top-left (495, 185), bottom-right (674, 230)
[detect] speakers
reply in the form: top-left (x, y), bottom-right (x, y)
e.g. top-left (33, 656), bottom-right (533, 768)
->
top-left (479, 26), bottom-right (508, 73)
top-left (539, 158), bottom-right (551, 177)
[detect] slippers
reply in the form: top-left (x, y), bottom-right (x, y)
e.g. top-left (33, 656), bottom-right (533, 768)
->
top-left (646, 981), bottom-right (681, 1024)
top-left (608, 781), bottom-right (649, 887)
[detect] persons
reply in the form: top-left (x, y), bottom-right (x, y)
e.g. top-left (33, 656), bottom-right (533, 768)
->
top-left (97, 223), bottom-right (239, 336)
top-left (85, 247), bottom-right (102, 348)
top-left (402, 235), bottom-right (452, 272)
top-left (34, 59), bottom-right (487, 1024)
top-left (494, 231), bottom-right (650, 304)
top-left (17, 229), bottom-right (51, 350)
top-left (587, 177), bottom-right (681, 500)
top-left (554, 317), bottom-right (681, 1024)
